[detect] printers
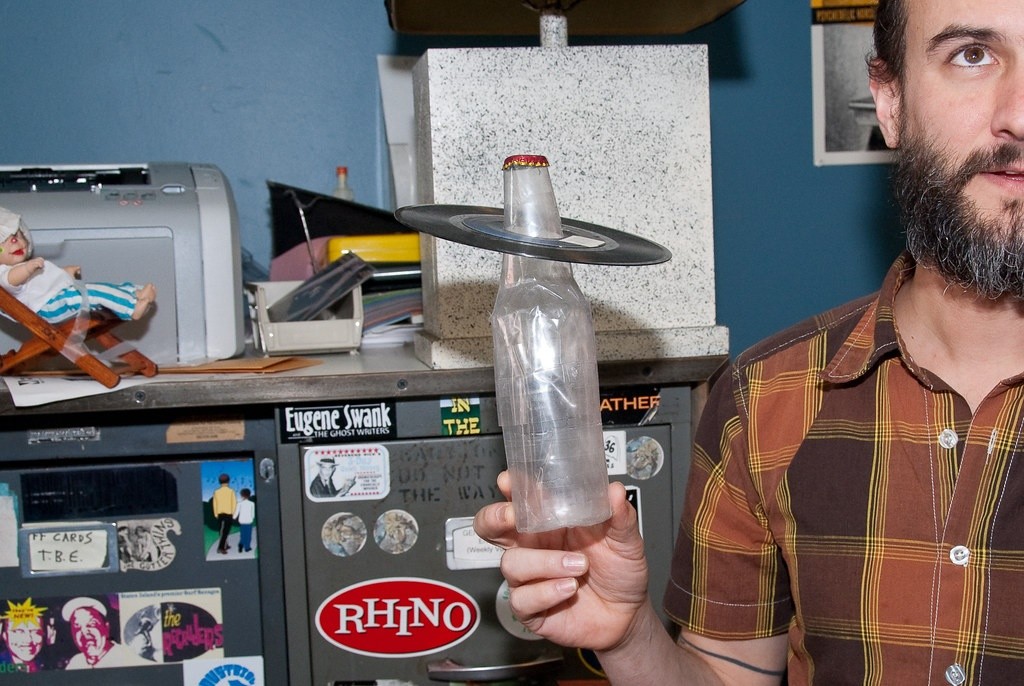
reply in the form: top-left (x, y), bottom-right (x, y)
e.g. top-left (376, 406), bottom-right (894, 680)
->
top-left (0, 160), bottom-right (246, 372)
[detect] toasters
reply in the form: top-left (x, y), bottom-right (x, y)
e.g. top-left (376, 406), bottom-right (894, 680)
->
top-left (0, 162), bottom-right (245, 369)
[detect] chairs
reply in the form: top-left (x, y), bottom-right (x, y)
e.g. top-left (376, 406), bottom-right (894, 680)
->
top-left (0, 287), bottom-right (158, 388)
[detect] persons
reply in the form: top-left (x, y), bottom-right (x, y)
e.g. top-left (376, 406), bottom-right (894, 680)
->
top-left (471, 0), bottom-right (1024, 686)
top-left (0, 207), bottom-right (156, 321)
top-left (0, 454), bottom-right (358, 673)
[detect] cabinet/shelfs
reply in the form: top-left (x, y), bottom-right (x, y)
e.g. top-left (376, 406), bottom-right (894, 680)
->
top-left (0, 343), bottom-right (727, 686)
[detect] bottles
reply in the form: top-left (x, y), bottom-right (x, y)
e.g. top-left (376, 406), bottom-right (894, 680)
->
top-left (330, 166), bottom-right (355, 202)
top-left (491, 154), bottom-right (612, 534)
